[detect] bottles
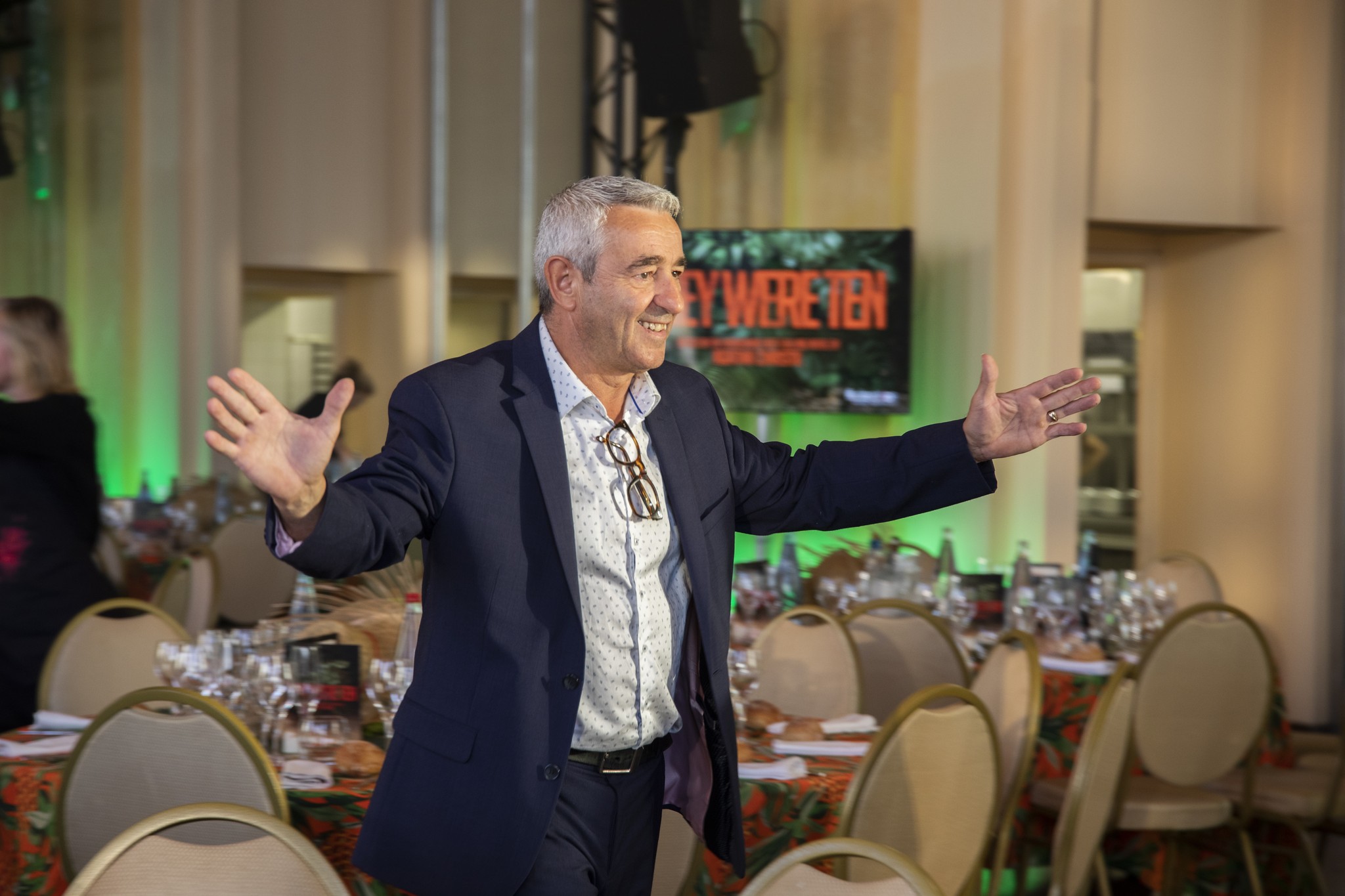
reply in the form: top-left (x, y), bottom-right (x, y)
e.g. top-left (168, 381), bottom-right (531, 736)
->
top-left (392, 589), bottom-right (422, 703)
top-left (776, 531), bottom-right (1031, 639)
top-left (291, 573), bottom-right (320, 624)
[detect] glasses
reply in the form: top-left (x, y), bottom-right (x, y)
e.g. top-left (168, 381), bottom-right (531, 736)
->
top-left (595, 420), bottom-right (664, 519)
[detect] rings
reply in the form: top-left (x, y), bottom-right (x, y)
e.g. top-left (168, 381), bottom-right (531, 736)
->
top-left (1046, 410), bottom-right (1058, 422)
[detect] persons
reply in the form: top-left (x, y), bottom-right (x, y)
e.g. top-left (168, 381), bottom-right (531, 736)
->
top-left (264, 357), bottom-right (376, 617)
top-left (204, 176), bottom-right (1101, 896)
top-left (0, 293), bottom-right (128, 736)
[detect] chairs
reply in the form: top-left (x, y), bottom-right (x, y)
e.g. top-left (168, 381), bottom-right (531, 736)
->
top-left (0, 479), bottom-right (1345, 895)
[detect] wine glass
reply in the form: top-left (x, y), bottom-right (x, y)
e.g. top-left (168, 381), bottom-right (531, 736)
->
top-left (1021, 569), bottom-right (1184, 663)
top-left (368, 657), bottom-right (412, 746)
top-left (729, 649), bottom-right (761, 741)
top-left (153, 618), bottom-right (343, 773)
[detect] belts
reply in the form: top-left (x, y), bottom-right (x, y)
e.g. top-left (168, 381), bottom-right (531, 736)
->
top-left (567, 732), bottom-right (673, 776)
